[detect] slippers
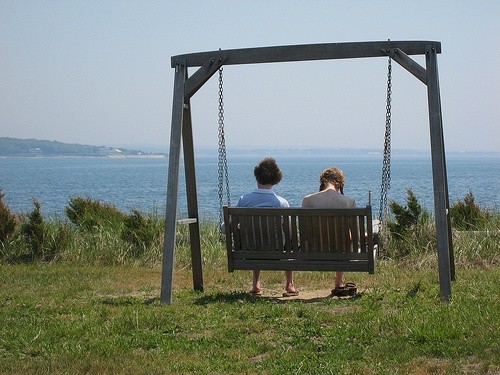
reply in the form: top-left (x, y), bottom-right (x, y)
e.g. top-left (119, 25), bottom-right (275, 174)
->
top-left (249, 290), bottom-right (262, 295)
top-left (283, 290), bottom-right (300, 296)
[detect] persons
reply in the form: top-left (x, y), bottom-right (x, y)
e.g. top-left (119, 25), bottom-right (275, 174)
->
top-left (302, 167), bottom-right (368, 289)
top-left (221, 157), bottom-right (297, 293)
